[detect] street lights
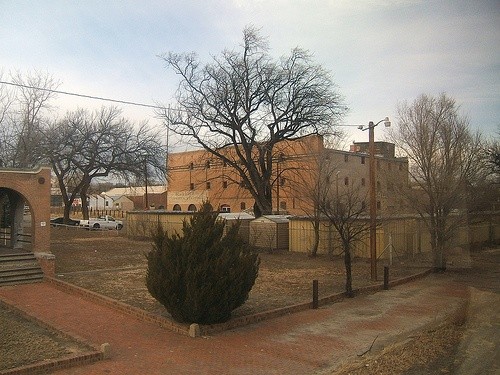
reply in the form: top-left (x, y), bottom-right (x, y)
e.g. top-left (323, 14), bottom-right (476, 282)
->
top-left (359, 116), bottom-right (391, 282)
top-left (276, 152), bottom-right (285, 215)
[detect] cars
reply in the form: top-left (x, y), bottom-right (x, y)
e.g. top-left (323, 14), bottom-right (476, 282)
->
top-left (50, 217), bottom-right (80, 226)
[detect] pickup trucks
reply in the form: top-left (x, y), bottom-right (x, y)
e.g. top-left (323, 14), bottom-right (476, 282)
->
top-left (79, 214), bottom-right (123, 231)
